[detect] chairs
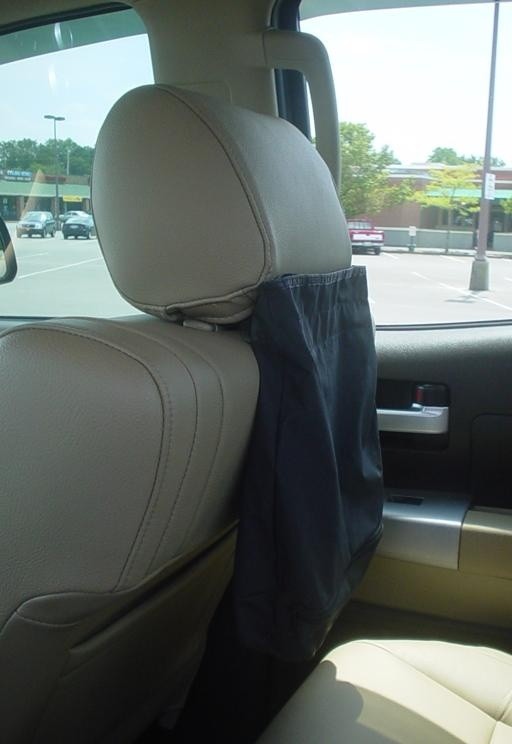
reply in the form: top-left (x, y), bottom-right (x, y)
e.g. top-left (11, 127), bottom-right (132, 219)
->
top-left (260, 637), bottom-right (511, 744)
top-left (0, 84), bottom-right (350, 743)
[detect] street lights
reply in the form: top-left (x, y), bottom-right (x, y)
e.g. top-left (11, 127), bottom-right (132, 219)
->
top-left (45, 115), bottom-right (65, 230)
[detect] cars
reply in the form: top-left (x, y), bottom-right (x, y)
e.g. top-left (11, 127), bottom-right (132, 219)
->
top-left (17, 211), bottom-right (56, 237)
top-left (59, 210), bottom-right (93, 240)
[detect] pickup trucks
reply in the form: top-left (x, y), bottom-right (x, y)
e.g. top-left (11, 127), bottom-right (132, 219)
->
top-left (347, 220), bottom-right (384, 254)
